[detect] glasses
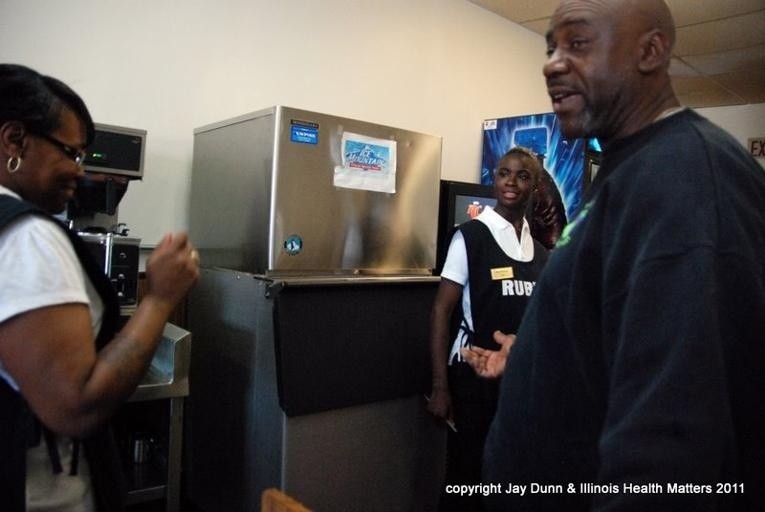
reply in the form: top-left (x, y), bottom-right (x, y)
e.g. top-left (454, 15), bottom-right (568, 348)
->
top-left (40, 133), bottom-right (86, 166)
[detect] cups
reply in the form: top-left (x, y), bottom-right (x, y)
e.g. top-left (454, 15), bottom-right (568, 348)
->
top-left (130, 433), bottom-right (152, 464)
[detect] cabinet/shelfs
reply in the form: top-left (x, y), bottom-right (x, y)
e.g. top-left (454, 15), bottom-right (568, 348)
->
top-left (120, 322), bottom-right (193, 512)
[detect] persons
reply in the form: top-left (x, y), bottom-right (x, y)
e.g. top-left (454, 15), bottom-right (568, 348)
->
top-left (431, 1), bottom-right (760, 509)
top-left (1, 64), bottom-right (200, 509)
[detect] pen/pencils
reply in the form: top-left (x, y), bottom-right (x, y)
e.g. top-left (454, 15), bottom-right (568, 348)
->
top-left (424, 395), bottom-right (457, 432)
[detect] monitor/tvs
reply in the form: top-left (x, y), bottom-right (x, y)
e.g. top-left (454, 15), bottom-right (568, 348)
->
top-left (445, 181), bottom-right (498, 231)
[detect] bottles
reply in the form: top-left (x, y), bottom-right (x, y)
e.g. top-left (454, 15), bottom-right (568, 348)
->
top-left (515, 127), bottom-right (568, 249)
